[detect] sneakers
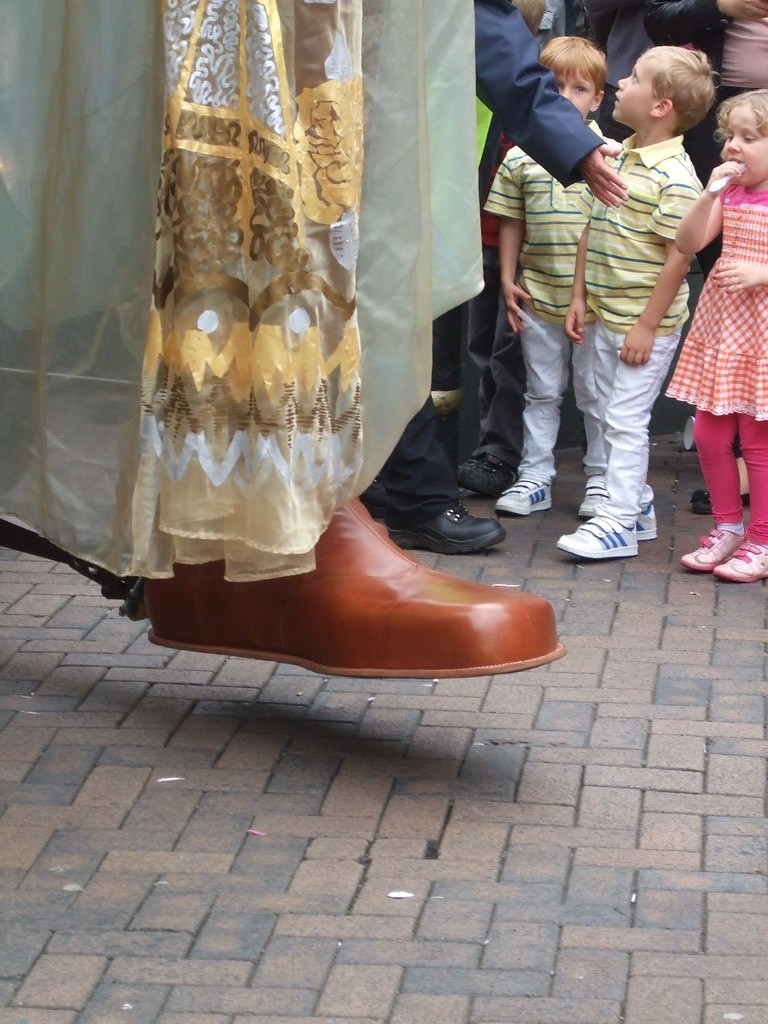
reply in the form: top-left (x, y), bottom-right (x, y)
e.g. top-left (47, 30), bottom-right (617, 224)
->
top-left (456, 455), bottom-right (512, 497)
top-left (360, 475), bottom-right (389, 518)
top-left (713, 539), bottom-right (768, 583)
top-left (680, 528), bottom-right (748, 572)
top-left (384, 498), bottom-right (505, 554)
top-left (577, 474), bottom-right (609, 516)
top-left (496, 471), bottom-right (554, 516)
top-left (635, 505), bottom-right (658, 540)
top-left (556, 513), bottom-right (639, 559)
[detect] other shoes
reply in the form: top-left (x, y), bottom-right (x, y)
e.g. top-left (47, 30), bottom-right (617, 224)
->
top-left (689, 489), bottom-right (751, 514)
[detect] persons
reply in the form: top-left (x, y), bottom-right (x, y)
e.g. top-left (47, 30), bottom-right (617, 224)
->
top-left (557, 46), bottom-right (716, 559)
top-left (456, 0), bottom-right (768, 496)
top-left (1, 0), bottom-right (567, 680)
top-left (483, 37), bottom-right (624, 519)
top-left (358, 0), bottom-right (627, 553)
top-left (665, 89), bottom-right (768, 581)
